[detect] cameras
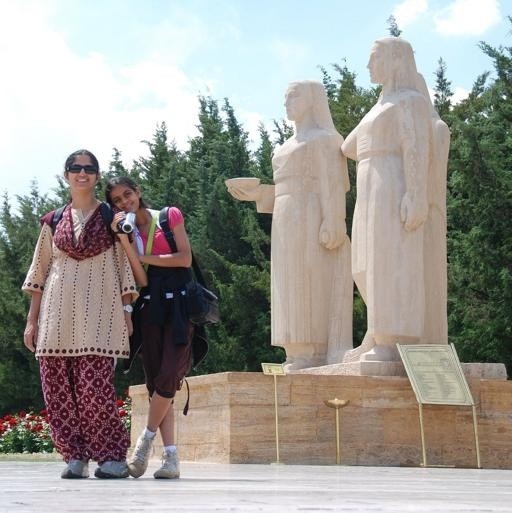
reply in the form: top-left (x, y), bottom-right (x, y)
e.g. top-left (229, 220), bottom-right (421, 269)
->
top-left (117, 212), bottom-right (137, 233)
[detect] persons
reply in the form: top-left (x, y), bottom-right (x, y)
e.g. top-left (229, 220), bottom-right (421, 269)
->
top-left (105, 175), bottom-right (220, 479)
top-left (226, 78), bottom-right (352, 372)
top-left (21, 148), bottom-right (141, 478)
top-left (343, 37), bottom-right (432, 359)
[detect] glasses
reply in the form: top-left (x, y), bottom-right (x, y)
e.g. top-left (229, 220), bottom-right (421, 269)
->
top-left (66, 164), bottom-right (98, 174)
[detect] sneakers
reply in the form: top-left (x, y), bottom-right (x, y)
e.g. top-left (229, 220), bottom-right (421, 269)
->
top-left (60, 460), bottom-right (89, 478)
top-left (94, 461), bottom-right (129, 477)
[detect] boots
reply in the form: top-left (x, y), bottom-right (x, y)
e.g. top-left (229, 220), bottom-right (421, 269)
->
top-left (128, 434), bottom-right (157, 478)
top-left (153, 450), bottom-right (180, 479)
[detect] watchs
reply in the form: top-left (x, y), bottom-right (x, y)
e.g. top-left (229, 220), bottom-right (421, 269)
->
top-left (124, 303), bottom-right (133, 312)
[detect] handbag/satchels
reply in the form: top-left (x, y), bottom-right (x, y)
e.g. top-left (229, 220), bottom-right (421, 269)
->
top-left (186, 279), bottom-right (220, 326)
